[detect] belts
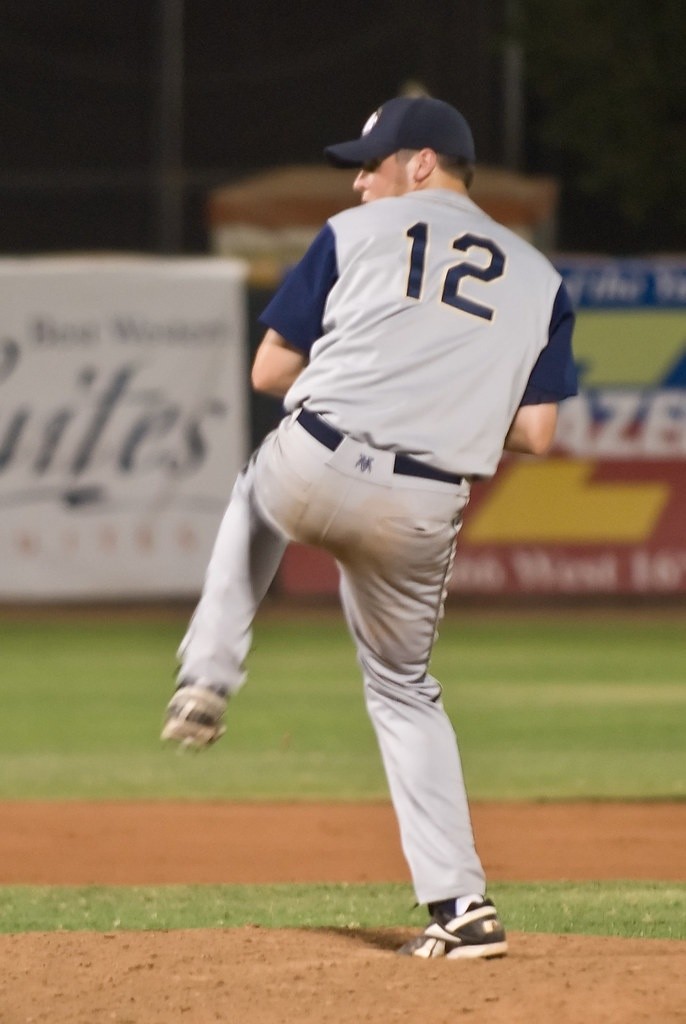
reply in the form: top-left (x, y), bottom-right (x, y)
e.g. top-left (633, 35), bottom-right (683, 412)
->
top-left (296, 409), bottom-right (461, 486)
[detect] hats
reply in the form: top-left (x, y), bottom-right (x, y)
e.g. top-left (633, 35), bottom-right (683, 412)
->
top-left (323, 93), bottom-right (475, 189)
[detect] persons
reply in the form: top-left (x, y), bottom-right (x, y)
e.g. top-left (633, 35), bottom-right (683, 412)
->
top-left (157, 93), bottom-right (580, 961)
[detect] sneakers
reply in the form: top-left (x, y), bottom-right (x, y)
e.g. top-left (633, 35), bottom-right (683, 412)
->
top-left (159, 677), bottom-right (230, 750)
top-left (397, 898), bottom-right (508, 959)
